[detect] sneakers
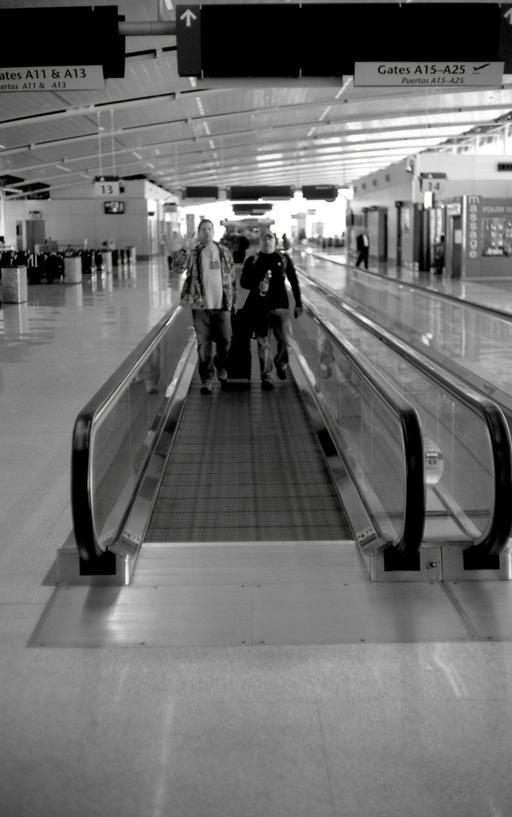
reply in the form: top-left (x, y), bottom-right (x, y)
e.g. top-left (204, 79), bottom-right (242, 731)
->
top-left (261, 382), bottom-right (274, 391)
top-left (275, 358), bottom-right (288, 380)
top-left (199, 378), bottom-right (213, 394)
top-left (213, 356), bottom-right (227, 381)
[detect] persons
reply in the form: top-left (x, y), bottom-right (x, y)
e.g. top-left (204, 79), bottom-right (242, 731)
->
top-left (167, 230), bottom-right (293, 272)
top-left (238, 224), bottom-right (304, 387)
top-left (0, 243), bottom-right (76, 284)
top-left (172, 218), bottom-right (241, 397)
top-left (354, 225), bottom-right (370, 268)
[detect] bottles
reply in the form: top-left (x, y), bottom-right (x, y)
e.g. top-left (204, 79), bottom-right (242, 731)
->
top-left (258, 270), bottom-right (274, 297)
